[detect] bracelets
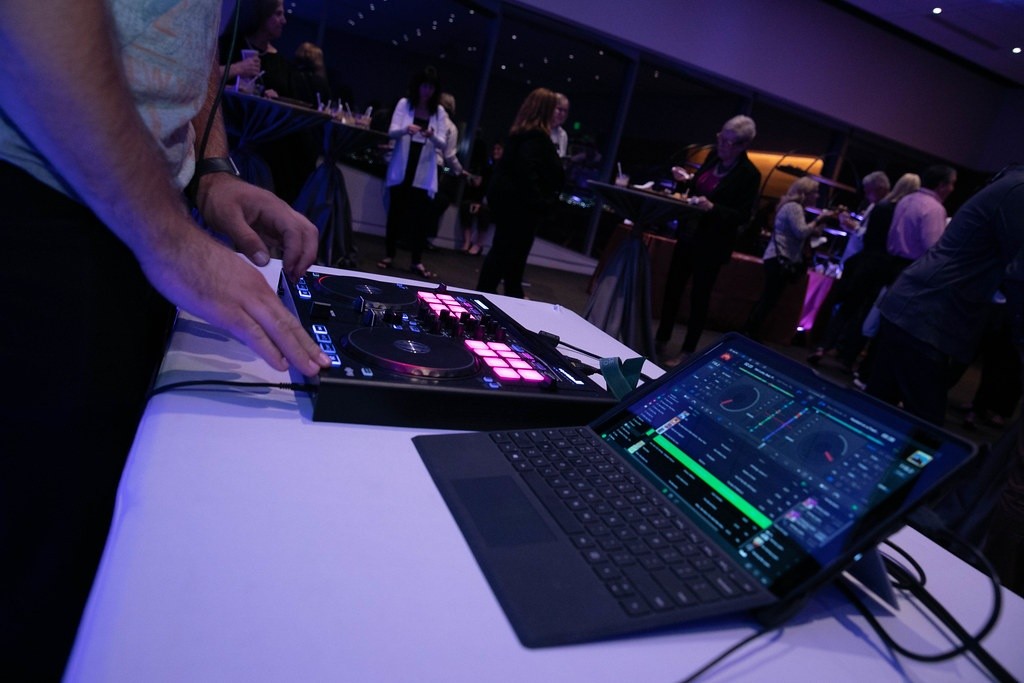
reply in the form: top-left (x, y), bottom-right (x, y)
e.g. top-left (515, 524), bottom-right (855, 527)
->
top-left (184, 155), bottom-right (241, 209)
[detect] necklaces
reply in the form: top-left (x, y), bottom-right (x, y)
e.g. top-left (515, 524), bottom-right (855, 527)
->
top-left (713, 158), bottom-right (740, 179)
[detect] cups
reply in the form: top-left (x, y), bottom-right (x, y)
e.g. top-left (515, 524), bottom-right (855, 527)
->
top-left (238, 78), bottom-right (255, 95)
top-left (324, 108), bottom-right (372, 130)
top-left (681, 193), bottom-right (688, 202)
top-left (241, 49), bottom-right (259, 62)
top-left (615, 173), bottom-right (629, 188)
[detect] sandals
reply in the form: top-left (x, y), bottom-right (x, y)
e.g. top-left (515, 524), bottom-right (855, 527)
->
top-left (411, 263), bottom-right (434, 278)
top-left (377, 259), bottom-right (392, 269)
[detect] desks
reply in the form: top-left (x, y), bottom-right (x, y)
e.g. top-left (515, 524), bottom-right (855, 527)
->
top-left (575, 179), bottom-right (706, 362)
top-left (60, 259), bottom-right (1024, 683)
top-left (226, 91), bottom-right (393, 275)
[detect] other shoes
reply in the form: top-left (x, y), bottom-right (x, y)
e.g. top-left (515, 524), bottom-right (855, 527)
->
top-left (653, 343), bottom-right (665, 353)
top-left (459, 242), bottom-right (473, 254)
top-left (664, 353), bottom-right (690, 367)
top-left (808, 351), bottom-right (823, 363)
top-left (465, 245), bottom-right (483, 255)
top-left (964, 412), bottom-right (976, 427)
top-left (989, 414), bottom-right (1004, 428)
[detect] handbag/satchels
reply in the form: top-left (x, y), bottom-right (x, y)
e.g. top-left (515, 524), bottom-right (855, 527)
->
top-left (766, 253), bottom-right (809, 283)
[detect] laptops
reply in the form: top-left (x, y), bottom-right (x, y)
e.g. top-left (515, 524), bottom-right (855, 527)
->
top-left (410, 330), bottom-right (982, 648)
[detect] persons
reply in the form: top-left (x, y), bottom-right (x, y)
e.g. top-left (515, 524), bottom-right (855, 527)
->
top-left (0, 0), bottom-right (354, 683)
top-left (653, 115), bottom-right (760, 367)
top-left (763, 154), bottom-right (1024, 599)
top-left (376, 68), bottom-right (472, 280)
top-left (460, 87), bottom-right (587, 299)
